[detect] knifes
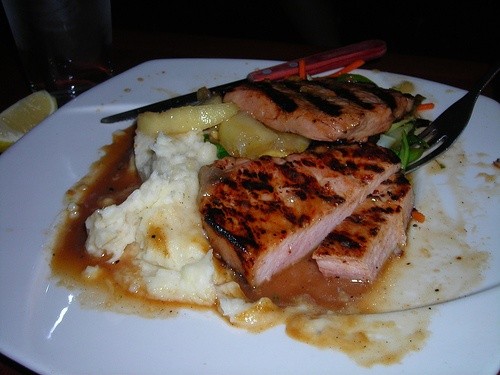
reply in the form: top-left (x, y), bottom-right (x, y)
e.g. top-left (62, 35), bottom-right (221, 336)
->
top-left (100, 40), bottom-right (387, 124)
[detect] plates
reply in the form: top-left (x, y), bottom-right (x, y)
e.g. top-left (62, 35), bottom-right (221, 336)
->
top-left (0, 58), bottom-right (500, 375)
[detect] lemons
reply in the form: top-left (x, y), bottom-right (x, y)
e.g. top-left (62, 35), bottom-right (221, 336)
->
top-left (0, 89), bottom-right (58, 153)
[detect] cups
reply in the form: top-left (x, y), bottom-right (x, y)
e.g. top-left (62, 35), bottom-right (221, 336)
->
top-left (1, 0), bottom-right (113, 106)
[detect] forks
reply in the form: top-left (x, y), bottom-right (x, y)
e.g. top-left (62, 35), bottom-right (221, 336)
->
top-left (391, 68), bottom-right (500, 174)
